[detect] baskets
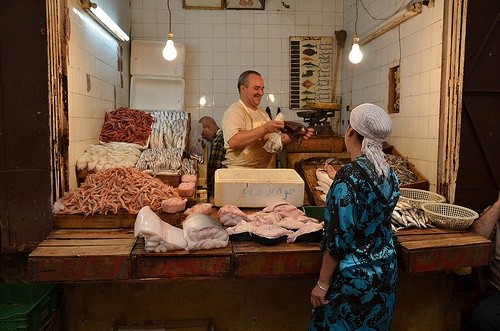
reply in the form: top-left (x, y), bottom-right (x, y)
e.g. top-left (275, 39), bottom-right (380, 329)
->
top-left (420, 202), bottom-right (479, 230)
top-left (388, 188), bottom-right (446, 206)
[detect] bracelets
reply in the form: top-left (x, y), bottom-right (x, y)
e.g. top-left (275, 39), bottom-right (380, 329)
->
top-left (317, 281), bottom-right (327, 292)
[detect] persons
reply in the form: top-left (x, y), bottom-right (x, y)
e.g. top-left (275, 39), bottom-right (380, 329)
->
top-left (308, 103), bottom-right (401, 331)
top-left (221, 70), bottom-right (314, 169)
top-left (197, 116), bottom-right (227, 194)
top-left (472, 195), bottom-right (500, 331)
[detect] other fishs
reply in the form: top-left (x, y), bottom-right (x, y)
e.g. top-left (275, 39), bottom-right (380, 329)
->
top-left (391, 201), bottom-right (435, 232)
top-left (139, 110), bottom-right (195, 175)
top-left (300, 42), bottom-right (321, 103)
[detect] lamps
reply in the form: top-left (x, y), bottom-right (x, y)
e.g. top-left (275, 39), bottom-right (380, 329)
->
top-left (82, 1), bottom-right (130, 42)
top-left (358, 2), bottom-right (423, 46)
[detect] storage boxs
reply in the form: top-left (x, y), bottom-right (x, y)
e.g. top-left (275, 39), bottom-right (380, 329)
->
top-left (0, 282), bottom-right (61, 331)
top-left (382, 147), bottom-right (428, 192)
top-left (214, 168), bottom-right (305, 208)
top-left (53, 39), bottom-right (192, 229)
